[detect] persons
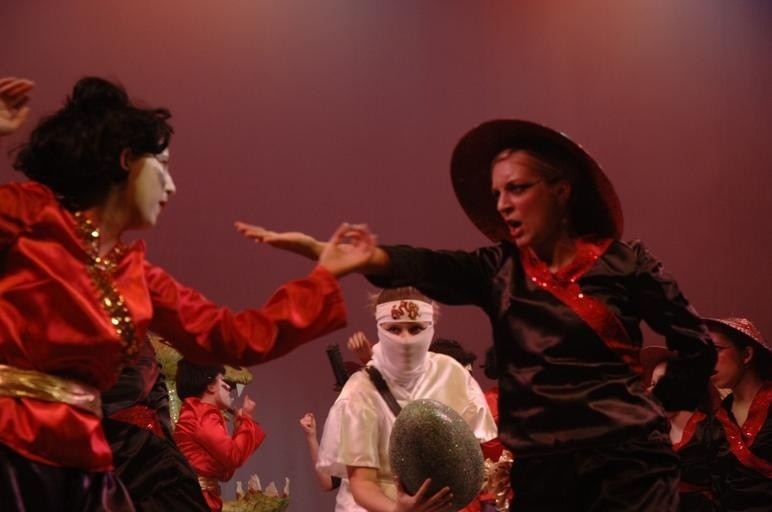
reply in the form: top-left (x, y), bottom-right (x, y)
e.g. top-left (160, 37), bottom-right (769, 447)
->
top-left (682, 309), bottom-right (771, 510)
top-left (1, 71), bottom-right (380, 512)
top-left (171, 363), bottom-right (264, 511)
top-left (236, 116), bottom-right (717, 512)
top-left (314, 281), bottom-right (499, 512)
top-left (298, 331), bottom-right (514, 511)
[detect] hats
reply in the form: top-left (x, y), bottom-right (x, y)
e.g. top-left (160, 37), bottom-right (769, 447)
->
top-left (698, 315), bottom-right (771, 382)
top-left (174, 357), bottom-right (225, 400)
top-left (639, 345), bottom-right (723, 416)
top-left (430, 338), bottom-right (477, 368)
top-left (450, 118), bottom-right (625, 243)
